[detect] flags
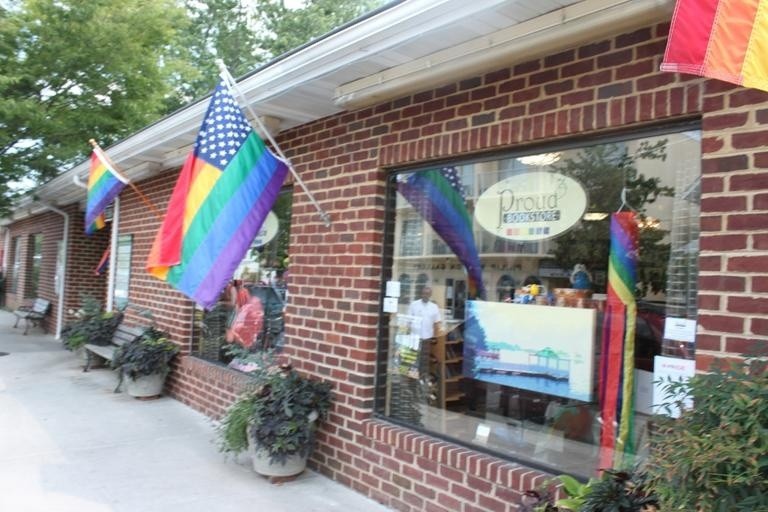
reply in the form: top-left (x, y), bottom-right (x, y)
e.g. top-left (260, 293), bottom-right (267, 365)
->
top-left (146, 73), bottom-right (290, 311)
top-left (660, 0), bottom-right (768, 92)
top-left (396, 166), bottom-right (486, 300)
top-left (85, 146), bottom-right (130, 235)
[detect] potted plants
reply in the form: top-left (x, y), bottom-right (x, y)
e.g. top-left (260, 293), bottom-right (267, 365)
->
top-left (212, 355), bottom-right (337, 484)
top-left (60, 291), bottom-right (118, 369)
top-left (105, 326), bottom-right (180, 402)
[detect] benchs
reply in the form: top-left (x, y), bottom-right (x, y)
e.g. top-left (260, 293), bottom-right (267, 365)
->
top-left (13, 296), bottom-right (52, 336)
top-left (83, 322), bottom-right (149, 394)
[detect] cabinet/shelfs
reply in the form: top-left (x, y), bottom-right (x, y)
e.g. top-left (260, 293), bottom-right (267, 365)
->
top-left (428, 282), bottom-right (467, 435)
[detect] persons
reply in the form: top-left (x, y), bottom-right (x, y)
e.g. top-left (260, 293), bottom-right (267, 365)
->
top-left (406, 287), bottom-right (442, 340)
top-left (218, 281), bottom-right (237, 308)
